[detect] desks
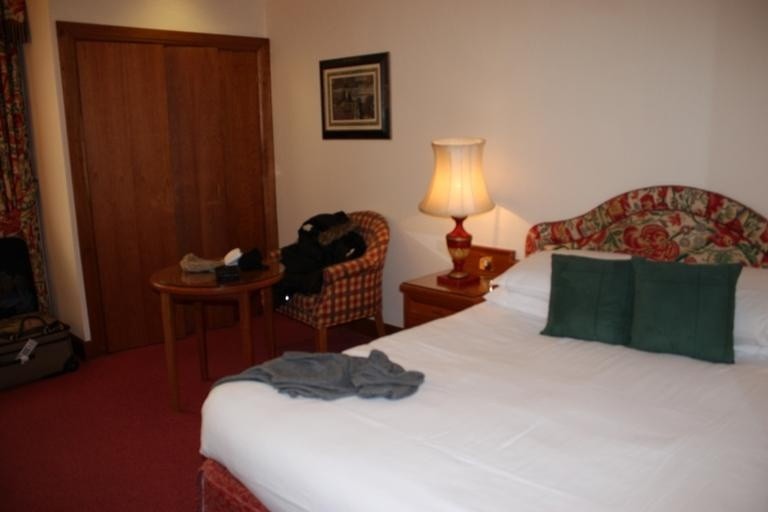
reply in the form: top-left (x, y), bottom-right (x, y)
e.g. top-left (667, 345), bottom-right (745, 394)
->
top-left (149, 266), bottom-right (282, 409)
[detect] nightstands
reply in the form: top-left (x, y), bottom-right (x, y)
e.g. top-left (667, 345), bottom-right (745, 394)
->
top-left (399, 246), bottom-right (518, 329)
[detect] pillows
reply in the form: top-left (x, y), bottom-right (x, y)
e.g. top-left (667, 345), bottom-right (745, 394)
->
top-left (482, 250), bottom-right (768, 364)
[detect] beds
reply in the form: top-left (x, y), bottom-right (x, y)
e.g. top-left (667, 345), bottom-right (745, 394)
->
top-left (200, 185), bottom-right (768, 512)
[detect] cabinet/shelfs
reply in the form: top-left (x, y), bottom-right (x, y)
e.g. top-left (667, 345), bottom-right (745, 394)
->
top-left (56, 21), bottom-right (279, 352)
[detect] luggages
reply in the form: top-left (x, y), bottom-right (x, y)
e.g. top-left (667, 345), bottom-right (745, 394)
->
top-left (1, 316), bottom-right (78, 389)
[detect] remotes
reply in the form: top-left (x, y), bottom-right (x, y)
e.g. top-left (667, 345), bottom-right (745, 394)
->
top-left (15, 338), bottom-right (38, 359)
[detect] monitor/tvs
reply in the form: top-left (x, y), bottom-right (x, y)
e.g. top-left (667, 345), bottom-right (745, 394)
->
top-left (0, 237), bottom-right (38, 320)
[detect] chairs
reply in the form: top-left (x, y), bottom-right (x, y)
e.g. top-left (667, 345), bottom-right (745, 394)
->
top-left (260, 211), bottom-right (390, 352)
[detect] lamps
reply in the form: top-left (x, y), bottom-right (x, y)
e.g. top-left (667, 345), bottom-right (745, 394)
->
top-left (418, 137), bottom-right (495, 290)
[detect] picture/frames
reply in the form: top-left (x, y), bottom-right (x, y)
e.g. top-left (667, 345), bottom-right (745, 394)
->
top-left (320, 52), bottom-right (390, 139)
